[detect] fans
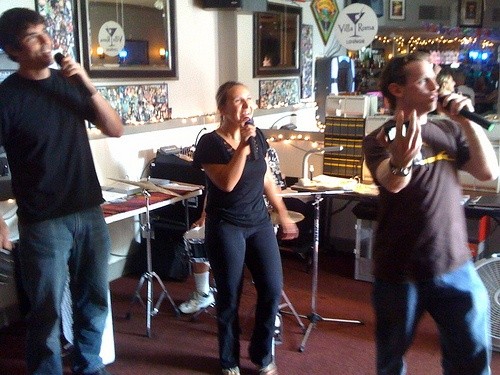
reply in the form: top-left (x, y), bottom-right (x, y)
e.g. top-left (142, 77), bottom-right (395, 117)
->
top-left (473, 252), bottom-right (500, 354)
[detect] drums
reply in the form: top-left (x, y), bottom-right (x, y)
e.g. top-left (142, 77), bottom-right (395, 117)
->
top-left (182, 225), bottom-right (207, 263)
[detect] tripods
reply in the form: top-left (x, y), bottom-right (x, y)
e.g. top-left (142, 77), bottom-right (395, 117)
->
top-left (277, 190), bottom-right (364, 351)
top-left (125, 189), bottom-right (180, 340)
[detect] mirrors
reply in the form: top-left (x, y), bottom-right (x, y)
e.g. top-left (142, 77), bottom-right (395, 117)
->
top-left (252, 2), bottom-right (303, 79)
top-left (73, 0), bottom-right (179, 82)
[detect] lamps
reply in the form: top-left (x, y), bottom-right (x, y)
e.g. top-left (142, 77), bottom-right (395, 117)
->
top-left (298, 145), bottom-right (343, 187)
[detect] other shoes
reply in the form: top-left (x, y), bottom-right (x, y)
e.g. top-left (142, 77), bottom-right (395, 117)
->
top-left (258, 362), bottom-right (278, 375)
top-left (222, 366), bottom-right (240, 375)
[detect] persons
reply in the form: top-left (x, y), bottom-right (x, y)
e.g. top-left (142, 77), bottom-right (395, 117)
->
top-left (194, 82), bottom-right (298, 375)
top-left (433, 63), bottom-right (500, 113)
top-left (262, 52), bottom-right (279, 67)
top-left (0, 7), bottom-right (123, 375)
top-left (363, 51), bottom-right (500, 375)
top-left (177, 117), bottom-right (285, 315)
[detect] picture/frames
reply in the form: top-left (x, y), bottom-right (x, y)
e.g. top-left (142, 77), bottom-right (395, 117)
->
top-left (388, 0), bottom-right (406, 21)
top-left (457, 0), bottom-right (484, 28)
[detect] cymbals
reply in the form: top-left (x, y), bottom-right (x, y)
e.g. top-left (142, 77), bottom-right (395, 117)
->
top-left (267, 208), bottom-right (305, 224)
top-left (107, 177), bottom-right (184, 198)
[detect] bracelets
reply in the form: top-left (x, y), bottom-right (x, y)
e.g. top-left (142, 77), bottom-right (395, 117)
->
top-left (86, 85), bottom-right (98, 96)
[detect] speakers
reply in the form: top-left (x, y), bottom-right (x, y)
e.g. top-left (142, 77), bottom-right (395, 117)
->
top-left (203, 0), bottom-right (242, 8)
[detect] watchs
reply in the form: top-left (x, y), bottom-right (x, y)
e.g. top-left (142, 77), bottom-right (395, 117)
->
top-left (389, 159), bottom-right (411, 176)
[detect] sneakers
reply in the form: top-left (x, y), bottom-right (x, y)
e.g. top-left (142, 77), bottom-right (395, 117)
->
top-left (178, 290), bottom-right (215, 313)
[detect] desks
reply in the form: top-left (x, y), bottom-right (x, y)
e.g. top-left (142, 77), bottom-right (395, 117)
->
top-left (101, 178), bottom-right (204, 227)
top-left (292, 176), bottom-right (500, 291)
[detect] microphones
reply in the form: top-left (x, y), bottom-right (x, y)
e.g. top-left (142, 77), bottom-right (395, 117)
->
top-left (194, 128), bottom-right (206, 146)
top-left (439, 96), bottom-right (495, 132)
top-left (270, 114), bottom-right (297, 130)
top-left (54, 53), bottom-right (93, 97)
top-left (244, 117), bottom-right (258, 161)
